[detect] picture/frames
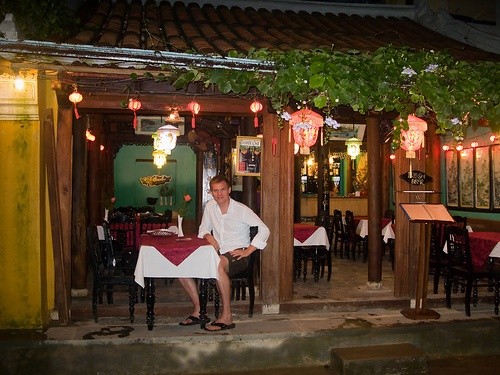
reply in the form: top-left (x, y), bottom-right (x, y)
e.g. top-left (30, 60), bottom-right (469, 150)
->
top-left (235, 136), bottom-right (262, 176)
top-left (445, 144), bottom-right (500, 214)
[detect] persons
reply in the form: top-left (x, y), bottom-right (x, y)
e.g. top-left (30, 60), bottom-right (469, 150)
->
top-left (178, 176), bottom-right (270, 331)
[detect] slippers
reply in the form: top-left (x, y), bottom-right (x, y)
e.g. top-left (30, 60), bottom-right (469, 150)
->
top-left (179, 316), bottom-right (210, 325)
top-left (204, 320), bottom-right (235, 331)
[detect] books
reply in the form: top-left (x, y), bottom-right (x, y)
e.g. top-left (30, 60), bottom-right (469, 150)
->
top-left (402, 203), bottom-right (454, 222)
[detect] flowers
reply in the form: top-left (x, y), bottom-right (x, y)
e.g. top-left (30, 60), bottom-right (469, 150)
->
top-left (178, 194), bottom-right (191, 218)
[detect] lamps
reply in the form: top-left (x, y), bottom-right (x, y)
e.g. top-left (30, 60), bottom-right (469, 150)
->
top-left (152, 122), bottom-right (178, 168)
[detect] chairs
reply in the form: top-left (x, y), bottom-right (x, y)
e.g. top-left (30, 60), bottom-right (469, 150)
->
top-left (88, 208), bottom-right (500, 331)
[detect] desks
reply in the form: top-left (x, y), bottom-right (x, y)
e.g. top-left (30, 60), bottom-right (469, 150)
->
top-left (293, 223), bottom-right (330, 283)
top-left (341, 215), bottom-right (396, 264)
top-left (454, 231), bottom-right (500, 308)
top-left (133, 233), bottom-right (221, 331)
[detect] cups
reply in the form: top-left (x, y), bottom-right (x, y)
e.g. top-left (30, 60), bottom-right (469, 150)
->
top-left (355, 191), bottom-right (360, 197)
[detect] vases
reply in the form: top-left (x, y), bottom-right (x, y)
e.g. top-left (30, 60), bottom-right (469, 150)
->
top-left (178, 214), bottom-right (184, 237)
